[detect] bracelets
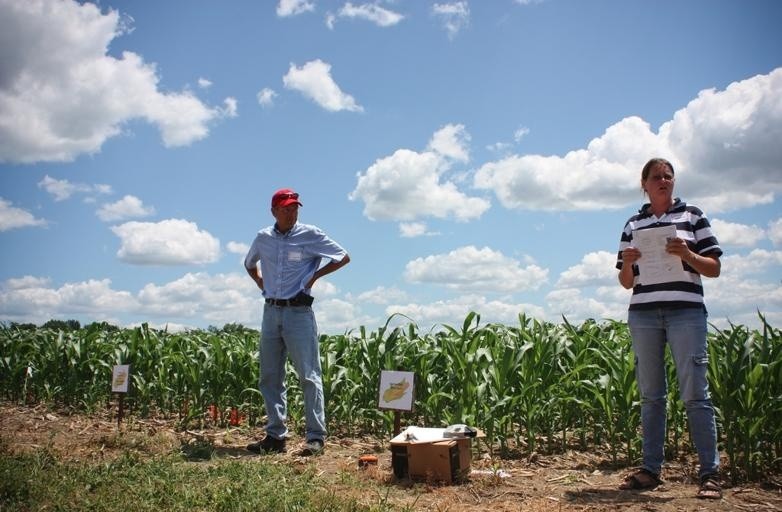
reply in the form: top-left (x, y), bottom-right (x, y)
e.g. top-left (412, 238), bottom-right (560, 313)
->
top-left (688, 252), bottom-right (697, 267)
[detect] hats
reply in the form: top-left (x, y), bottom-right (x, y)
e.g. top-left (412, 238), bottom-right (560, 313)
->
top-left (272, 190), bottom-right (304, 210)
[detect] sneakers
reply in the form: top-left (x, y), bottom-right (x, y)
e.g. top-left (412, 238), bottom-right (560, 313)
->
top-left (247, 434), bottom-right (287, 455)
top-left (302, 439), bottom-right (326, 455)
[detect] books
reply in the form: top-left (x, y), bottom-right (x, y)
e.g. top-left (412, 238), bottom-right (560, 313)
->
top-left (630, 224), bottom-right (685, 286)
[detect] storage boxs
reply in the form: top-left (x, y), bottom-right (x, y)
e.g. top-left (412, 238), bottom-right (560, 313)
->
top-left (389, 426), bottom-right (486, 483)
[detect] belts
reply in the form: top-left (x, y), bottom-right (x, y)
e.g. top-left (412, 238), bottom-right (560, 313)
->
top-left (264, 298), bottom-right (308, 308)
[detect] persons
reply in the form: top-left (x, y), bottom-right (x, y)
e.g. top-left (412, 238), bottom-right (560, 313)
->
top-left (244, 190), bottom-right (349, 456)
top-left (618, 156), bottom-right (722, 497)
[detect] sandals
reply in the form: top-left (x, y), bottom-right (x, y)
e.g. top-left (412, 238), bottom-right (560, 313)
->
top-left (618, 470), bottom-right (664, 490)
top-left (695, 476), bottom-right (722, 498)
